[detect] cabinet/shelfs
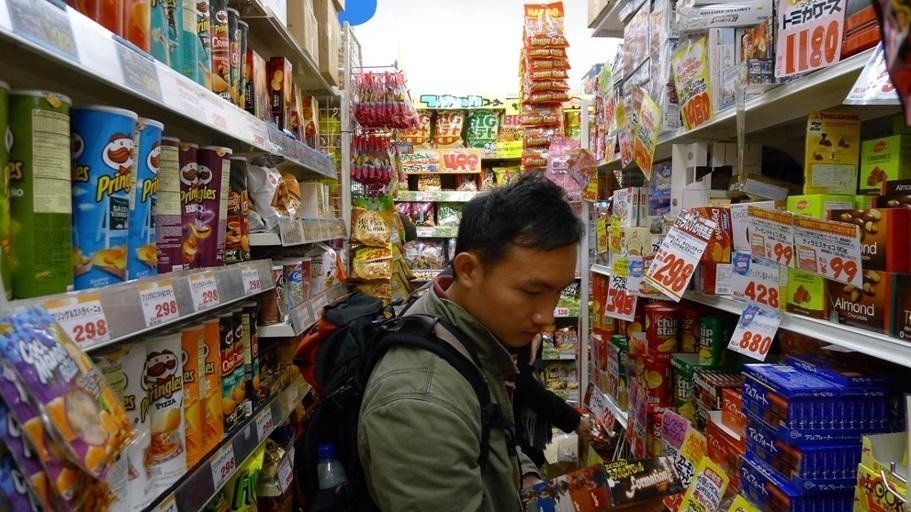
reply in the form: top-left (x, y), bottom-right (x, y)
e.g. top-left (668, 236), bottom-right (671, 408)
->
top-left (584, 0), bottom-right (909, 509)
top-left (316, 0), bottom-right (349, 86)
top-left (401, 106), bottom-right (589, 411)
top-left (1, 2), bottom-right (368, 512)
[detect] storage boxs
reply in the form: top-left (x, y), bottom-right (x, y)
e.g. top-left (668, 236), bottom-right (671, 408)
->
top-left (286, 0), bottom-right (318, 70)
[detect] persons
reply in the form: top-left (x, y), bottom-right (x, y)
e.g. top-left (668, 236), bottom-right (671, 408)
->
top-left (355, 168), bottom-right (586, 512)
top-left (529, 330), bottom-right (544, 382)
top-left (511, 343), bottom-right (592, 468)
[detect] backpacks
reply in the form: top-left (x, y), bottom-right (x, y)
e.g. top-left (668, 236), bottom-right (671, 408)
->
top-left (292, 293), bottom-right (514, 512)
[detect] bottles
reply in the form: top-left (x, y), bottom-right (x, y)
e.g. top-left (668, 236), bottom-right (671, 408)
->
top-left (313, 439), bottom-right (348, 489)
